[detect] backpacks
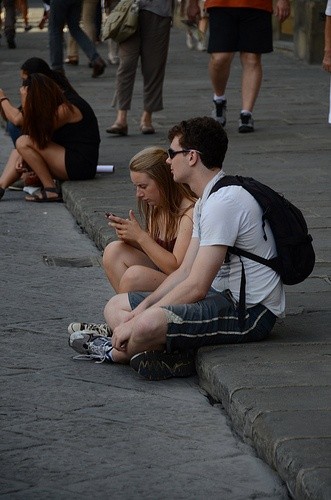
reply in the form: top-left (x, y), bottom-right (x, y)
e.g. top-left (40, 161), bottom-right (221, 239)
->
top-left (206, 175), bottom-right (316, 330)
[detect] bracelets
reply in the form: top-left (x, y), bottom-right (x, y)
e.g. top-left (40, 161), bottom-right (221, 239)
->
top-left (1, 98), bottom-right (10, 104)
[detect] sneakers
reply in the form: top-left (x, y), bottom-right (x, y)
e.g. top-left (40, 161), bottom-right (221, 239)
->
top-left (68, 330), bottom-right (127, 364)
top-left (210, 96), bottom-right (227, 127)
top-left (67, 322), bottom-right (114, 339)
top-left (130, 350), bottom-right (197, 381)
top-left (238, 112), bottom-right (255, 133)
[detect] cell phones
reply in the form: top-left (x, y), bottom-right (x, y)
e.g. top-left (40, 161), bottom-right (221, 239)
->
top-left (106, 212), bottom-right (122, 224)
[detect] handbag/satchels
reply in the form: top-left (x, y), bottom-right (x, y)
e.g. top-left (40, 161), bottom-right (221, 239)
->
top-left (101, 0), bottom-right (139, 43)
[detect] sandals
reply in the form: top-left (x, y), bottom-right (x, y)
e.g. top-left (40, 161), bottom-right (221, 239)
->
top-left (24, 186), bottom-right (64, 202)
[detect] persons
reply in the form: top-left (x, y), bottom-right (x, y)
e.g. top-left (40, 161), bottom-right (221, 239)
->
top-left (0, 57), bottom-right (101, 202)
top-left (1, 0), bottom-right (331, 134)
top-left (67, 117), bottom-right (287, 379)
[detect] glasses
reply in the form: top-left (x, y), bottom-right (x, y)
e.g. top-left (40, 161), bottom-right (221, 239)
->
top-left (167, 148), bottom-right (201, 159)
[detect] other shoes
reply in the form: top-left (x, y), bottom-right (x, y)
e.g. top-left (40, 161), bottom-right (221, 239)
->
top-left (7, 40), bottom-right (16, 49)
top-left (185, 32), bottom-right (194, 49)
top-left (108, 58), bottom-right (119, 65)
top-left (106, 122), bottom-right (128, 136)
top-left (197, 41), bottom-right (208, 51)
top-left (91, 58), bottom-right (107, 78)
top-left (8, 179), bottom-right (25, 190)
top-left (140, 123), bottom-right (154, 134)
top-left (25, 25), bottom-right (32, 31)
top-left (63, 56), bottom-right (79, 65)
